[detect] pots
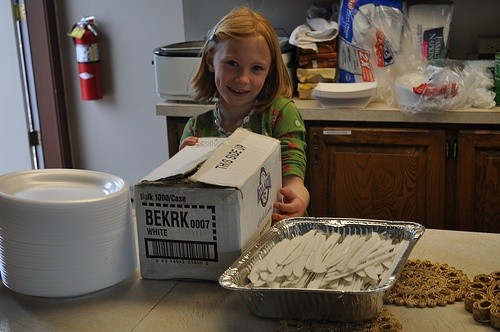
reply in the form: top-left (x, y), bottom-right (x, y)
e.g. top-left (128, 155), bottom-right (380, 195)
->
top-left (152, 39), bottom-right (294, 101)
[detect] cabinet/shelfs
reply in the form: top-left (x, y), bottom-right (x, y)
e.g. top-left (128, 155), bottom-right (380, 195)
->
top-left (156, 99), bottom-right (499, 233)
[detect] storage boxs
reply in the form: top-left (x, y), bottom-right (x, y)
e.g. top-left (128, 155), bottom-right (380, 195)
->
top-left (134, 127), bottom-right (283, 281)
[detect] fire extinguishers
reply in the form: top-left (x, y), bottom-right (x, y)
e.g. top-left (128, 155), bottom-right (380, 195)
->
top-left (68, 16), bottom-right (102, 101)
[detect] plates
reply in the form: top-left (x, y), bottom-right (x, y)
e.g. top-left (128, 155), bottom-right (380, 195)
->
top-left (313, 82), bottom-right (378, 109)
top-left (0, 168), bottom-right (136, 297)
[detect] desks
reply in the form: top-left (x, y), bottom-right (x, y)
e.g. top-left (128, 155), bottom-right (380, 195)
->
top-left (0, 229), bottom-right (500, 332)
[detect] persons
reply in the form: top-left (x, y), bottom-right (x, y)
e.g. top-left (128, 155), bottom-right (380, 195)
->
top-left (178, 5), bottom-right (310, 226)
